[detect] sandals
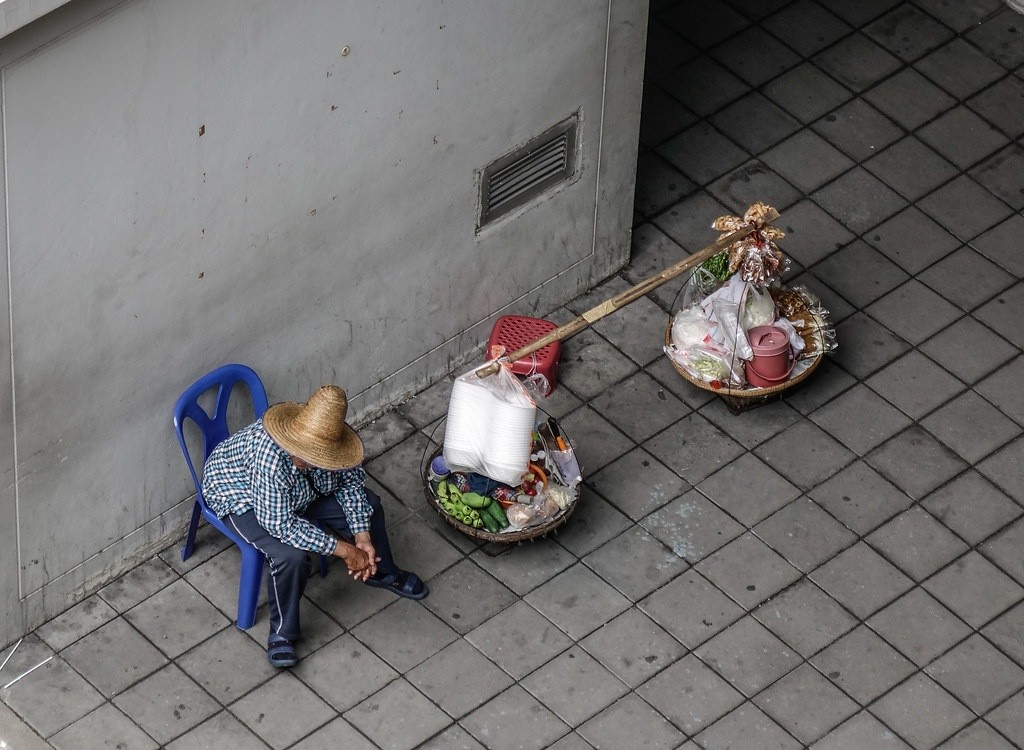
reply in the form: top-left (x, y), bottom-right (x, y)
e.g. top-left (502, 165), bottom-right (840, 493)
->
top-left (362, 564), bottom-right (428, 600)
top-left (268, 634), bottom-right (297, 666)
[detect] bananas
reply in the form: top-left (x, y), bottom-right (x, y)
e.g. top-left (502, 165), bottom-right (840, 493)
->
top-left (440, 479), bottom-right (483, 528)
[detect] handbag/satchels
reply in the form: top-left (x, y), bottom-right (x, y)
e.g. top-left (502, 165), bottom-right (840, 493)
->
top-left (663, 344), bottom-right (745, 389)
top-left (683, 265), bottom-right (731, 310)
top-left (442, 358), bottom-right (536, 488)
top-left (743, 283), bottom-right (775, 330)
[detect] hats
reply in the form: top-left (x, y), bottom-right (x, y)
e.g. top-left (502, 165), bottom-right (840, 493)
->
top-left (262, 385), bottom-right (365, 471)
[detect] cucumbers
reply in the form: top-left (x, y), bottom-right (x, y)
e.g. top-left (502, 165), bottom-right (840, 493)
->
top-left (478, 498), bottom-right (509, 533)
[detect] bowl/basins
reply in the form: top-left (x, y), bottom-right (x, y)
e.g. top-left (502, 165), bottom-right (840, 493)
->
top-left (443, 378), bottom-right (536, 486)
top-left (497, 464), bottom-right (548, 508)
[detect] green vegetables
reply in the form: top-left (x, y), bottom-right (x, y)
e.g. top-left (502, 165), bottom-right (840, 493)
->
top-left (700, 250), bottom-right (730, 285)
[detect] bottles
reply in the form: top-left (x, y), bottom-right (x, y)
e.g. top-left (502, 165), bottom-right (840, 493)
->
top-left (492, 486), bottom-right (534, 504)
top-left (530, 432), bottom-right (545, 470)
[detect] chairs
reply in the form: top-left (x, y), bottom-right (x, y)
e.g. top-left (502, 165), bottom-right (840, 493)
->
top-left (172, 363), bottom-right (328, 633)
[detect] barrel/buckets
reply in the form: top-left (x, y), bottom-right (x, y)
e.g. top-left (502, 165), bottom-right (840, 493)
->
top-left (745, 326), bottom-right (795, 388)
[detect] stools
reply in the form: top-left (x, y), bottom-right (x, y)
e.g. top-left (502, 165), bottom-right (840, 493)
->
top-left (485, 316), bottom-right (562, 396)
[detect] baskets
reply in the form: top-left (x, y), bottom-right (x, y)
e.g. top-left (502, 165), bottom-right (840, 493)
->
top-left (424, 440), bottom-right (580, 557)
top-left (664, 283), bottom-right (826, 415)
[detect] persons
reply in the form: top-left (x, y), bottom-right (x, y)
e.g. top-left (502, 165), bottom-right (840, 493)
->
top-left (201, 385), bottom-right (429, 667)
